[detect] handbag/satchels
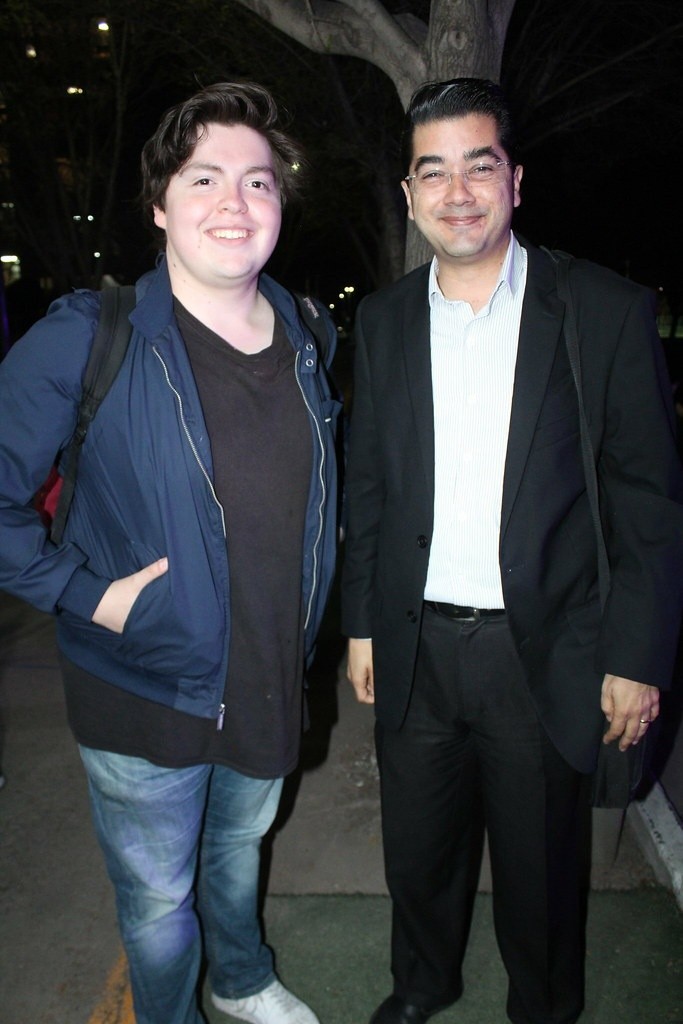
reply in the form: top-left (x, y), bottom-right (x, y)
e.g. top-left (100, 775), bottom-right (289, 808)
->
top-left (590, 619), bottom-right (683, 809)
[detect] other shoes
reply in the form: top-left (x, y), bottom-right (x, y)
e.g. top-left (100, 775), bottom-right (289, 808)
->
top-left (369, 977), bottom-right (464, 1024)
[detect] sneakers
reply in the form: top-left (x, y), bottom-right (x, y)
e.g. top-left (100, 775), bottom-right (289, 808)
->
top-left (210, 971), bottom-right (319, 1024)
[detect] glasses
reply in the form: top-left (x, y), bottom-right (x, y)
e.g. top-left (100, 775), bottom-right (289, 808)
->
top-left (405, 161), bottom-right (510, 186)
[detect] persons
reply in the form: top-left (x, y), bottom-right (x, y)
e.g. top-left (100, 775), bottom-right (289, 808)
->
top-left (0, 80), bottom-right (343, 1024)
top-left (344, 78), bottom-right (683, 1024)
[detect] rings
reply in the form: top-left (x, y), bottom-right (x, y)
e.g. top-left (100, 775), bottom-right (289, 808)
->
top-left (640, 720), bottom-right (651, 723)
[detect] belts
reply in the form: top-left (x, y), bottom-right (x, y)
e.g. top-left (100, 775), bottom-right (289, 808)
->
top-left (423, 601), bottom-right (506, 618)
